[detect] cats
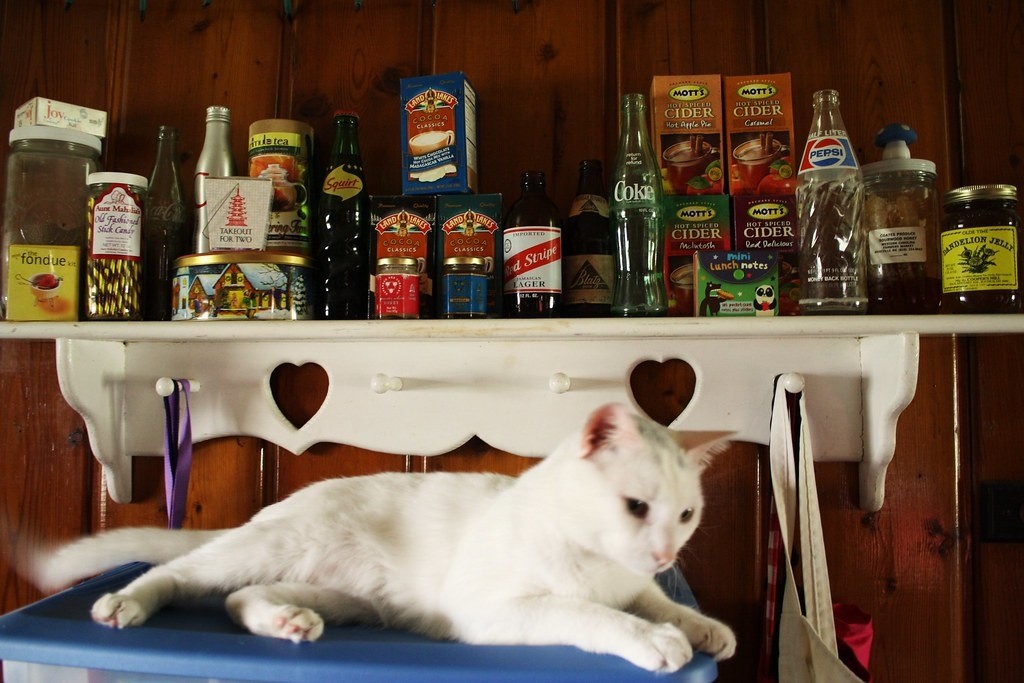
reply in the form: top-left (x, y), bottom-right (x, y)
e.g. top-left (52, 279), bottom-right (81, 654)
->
top-left (37, 404), bottom-right (738, 674)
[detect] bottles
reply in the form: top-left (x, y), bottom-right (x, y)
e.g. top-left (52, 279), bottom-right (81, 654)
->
top-left (795, 89), bottom-right (868, 314)
top-left (613, 94), bottom-right (669, 317)
top-left (564, 160), bottom-right (614, 318)
top-left (139, 126), bottom-right (188, 321)
top-left (315, 110), bottom-right (375, 320)
top-left (502, 171), bottom-right (563, 318)
top-left (192, 106), bottom-right (235, 255)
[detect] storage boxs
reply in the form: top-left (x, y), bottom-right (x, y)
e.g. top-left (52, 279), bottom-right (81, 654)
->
top-left (1, 558), bottom-right (718, 683)
top-left (366, 69), bottom-right (503, 321)
top-left (14, 96), bottom-right (107, 138)
top-left (648, 72), bottom-right (798, 317)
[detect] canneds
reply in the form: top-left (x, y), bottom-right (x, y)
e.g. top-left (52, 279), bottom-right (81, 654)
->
top-left (441, 257), bottom-right (488, 320)
top-left (375, 256), bottom-right (420, 321)
top-left (940, 183), bottom-right (1023, 315)
top-left (860, 159), bottom-right (942, 314)
